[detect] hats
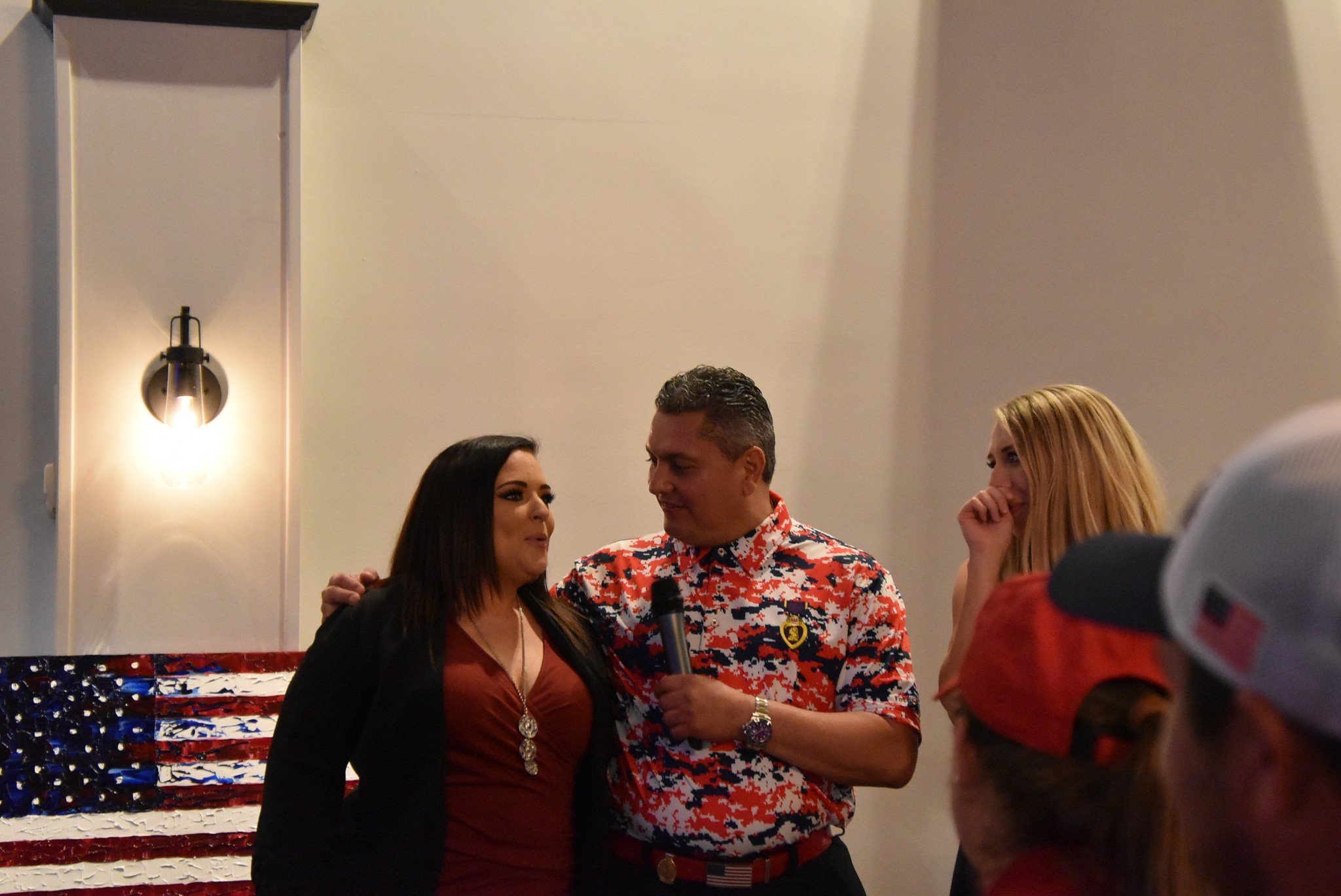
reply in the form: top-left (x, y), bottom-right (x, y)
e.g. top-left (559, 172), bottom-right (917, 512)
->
top-left (1045, 395), bottom-right (1341, 738)
top-left (934, 569), bottom-right (1173, 760)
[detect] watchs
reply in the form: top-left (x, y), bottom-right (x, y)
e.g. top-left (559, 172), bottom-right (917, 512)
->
top-left (743, 697), bottom-right (772, 752)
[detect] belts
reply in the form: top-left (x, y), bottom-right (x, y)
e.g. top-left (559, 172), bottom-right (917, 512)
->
top-left (609, 827), bottom-right (832, 889)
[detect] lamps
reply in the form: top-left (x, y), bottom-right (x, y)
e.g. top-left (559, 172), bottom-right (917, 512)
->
top-left (142, 306), bottom-right (229, 431)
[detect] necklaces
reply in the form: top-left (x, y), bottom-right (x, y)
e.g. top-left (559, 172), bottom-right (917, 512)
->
top-left (463, 595), bottom-right (538, 775)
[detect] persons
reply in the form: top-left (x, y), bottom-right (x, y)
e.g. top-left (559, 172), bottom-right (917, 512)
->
top-left (251, 435), bottom-right (613, 896)
top-left (933, 385), bottom-right (1341, 895)
top-left (321, 363), bottom-right (921, 896)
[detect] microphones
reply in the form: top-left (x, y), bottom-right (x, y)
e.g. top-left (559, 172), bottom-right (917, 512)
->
top-left (649, 577), bottom-right (703, 752)
